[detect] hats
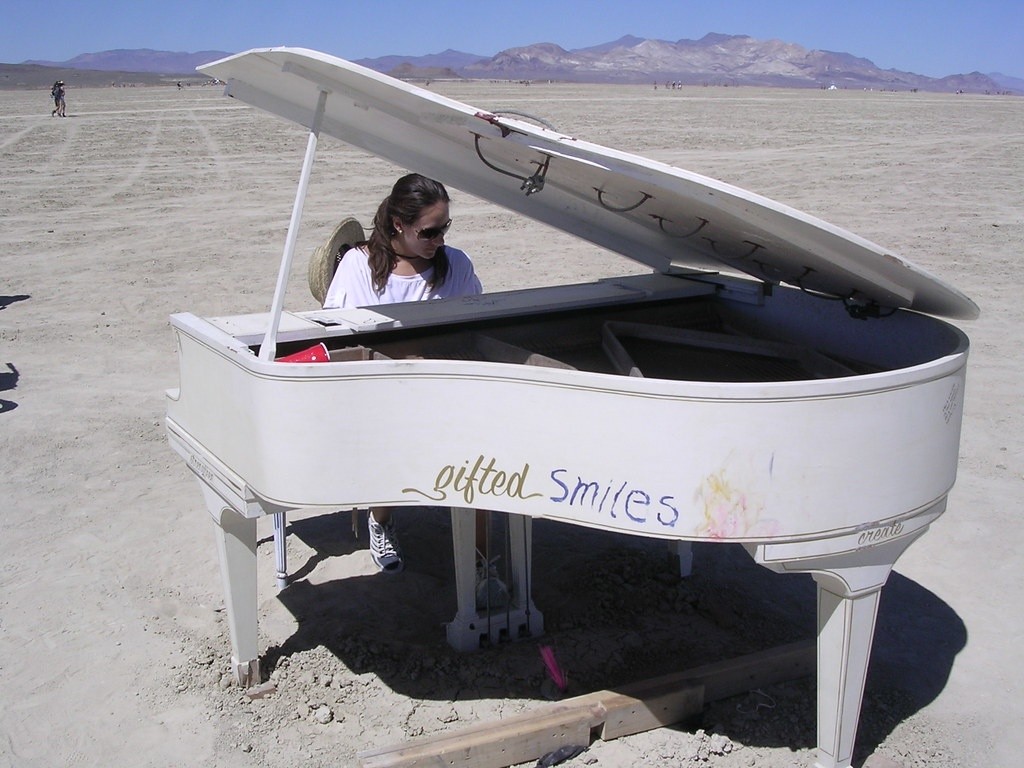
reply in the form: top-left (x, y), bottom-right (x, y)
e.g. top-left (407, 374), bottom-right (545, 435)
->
top-left (308, 216), bottom-right (365, 307)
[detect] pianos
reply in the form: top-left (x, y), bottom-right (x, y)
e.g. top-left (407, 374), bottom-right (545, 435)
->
top-left (160, 44), bottom-right (981, 768)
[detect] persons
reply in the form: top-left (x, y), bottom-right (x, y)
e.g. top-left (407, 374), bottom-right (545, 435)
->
top-left (653, 81), bottom-right (657, 89)
top-left (177, 82), bottom-right (181, 90)
top-left (52, 80), bottom-right (66, 117)
top-left (666, 81), bottom-right (682, 89)
top-left (324, 173), bottom-right (479, 308)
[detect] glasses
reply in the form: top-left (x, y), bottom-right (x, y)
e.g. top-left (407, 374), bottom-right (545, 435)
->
top-left (414, 218), bottom-right (454, 241)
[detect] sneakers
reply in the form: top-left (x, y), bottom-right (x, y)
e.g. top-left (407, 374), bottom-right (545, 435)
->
top-left (368, 508), bottom-right (404, 573)
top-left (473, 547), bottom-right (511, 608)
